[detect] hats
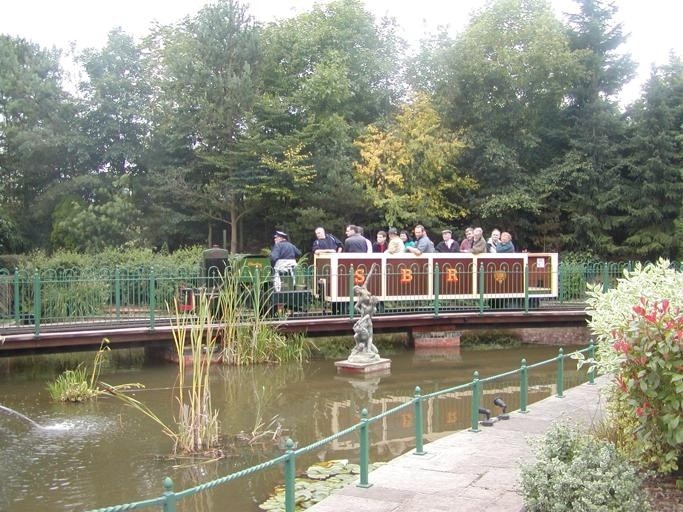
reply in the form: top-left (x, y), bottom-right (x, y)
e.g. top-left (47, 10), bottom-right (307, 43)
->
top-left (272, 230), bottom-right (289, 239)
top-left (440, 229), bottom-right (453, 234)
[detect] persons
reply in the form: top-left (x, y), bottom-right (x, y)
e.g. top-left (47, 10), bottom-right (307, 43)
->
top-left (310, 224), bottom-right (515, 254)
top-left (269, 230), bottom-right (301, 293)
top-left (351, 261), bottom-right (380, 353)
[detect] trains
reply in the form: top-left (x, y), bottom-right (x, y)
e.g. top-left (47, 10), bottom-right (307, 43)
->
top-left (171, 246), bottom-right (560, 318)
